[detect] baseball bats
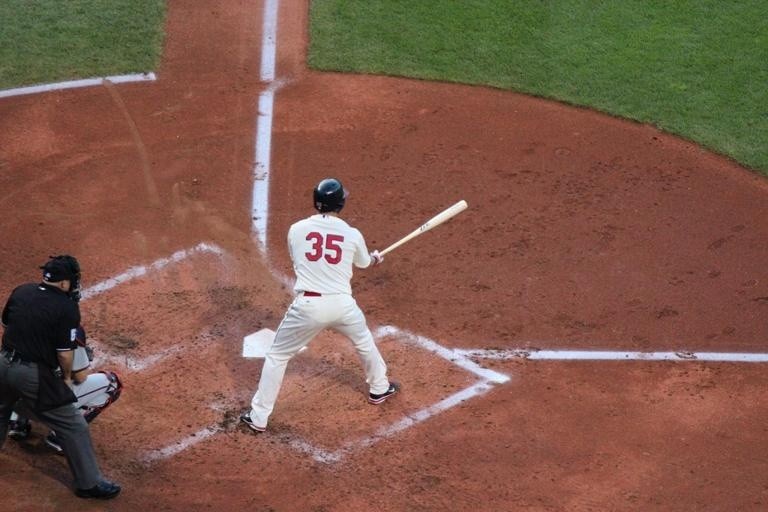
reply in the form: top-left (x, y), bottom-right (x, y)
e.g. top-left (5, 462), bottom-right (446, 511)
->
top-left (378, 199), bottom-right (469, 258)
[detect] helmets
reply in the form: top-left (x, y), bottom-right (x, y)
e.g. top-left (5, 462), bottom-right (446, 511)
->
top-left (43, 254), bottom-right (81, 300)
top-left (314, 178), bottom-right (348, 203)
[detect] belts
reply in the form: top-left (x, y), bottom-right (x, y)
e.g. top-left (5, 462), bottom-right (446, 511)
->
top-left (303, 290), bottom-right (320, 297)
top-left (0, 349), bottom-right (29, 366)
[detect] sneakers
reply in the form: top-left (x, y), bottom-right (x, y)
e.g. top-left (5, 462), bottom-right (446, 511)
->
top-left (241, 411), bottom-right (266, 432)
top-left (76, 482), bottom-right (120, 499)
top-left (44, 432), bottom-right (62, 452)
top-left (6, 421), bottom-right (32, 436)
top-left (368, 382), bottom-right (397, 404)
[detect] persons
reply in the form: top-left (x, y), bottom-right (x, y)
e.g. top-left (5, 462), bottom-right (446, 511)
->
top-left (240, 178), bottom-right (400, 433)
top-left (0, 254), bottom-right (121, 499)
top-left (8, 288), bottom-right (124, 455)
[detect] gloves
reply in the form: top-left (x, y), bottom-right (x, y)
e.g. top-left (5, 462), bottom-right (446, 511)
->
top-left (370, 250), bottom-right (383, 266)
